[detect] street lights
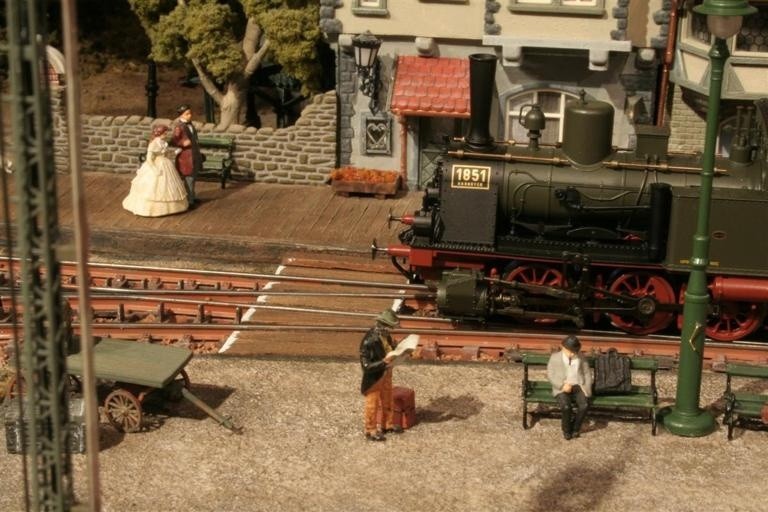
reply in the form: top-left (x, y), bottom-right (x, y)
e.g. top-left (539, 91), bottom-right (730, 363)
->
top-left (656, 0), bottom-right (758, 438)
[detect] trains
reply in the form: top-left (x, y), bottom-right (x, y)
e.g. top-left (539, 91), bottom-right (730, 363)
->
top-left (368, 88), bottom-right (768, 343)
